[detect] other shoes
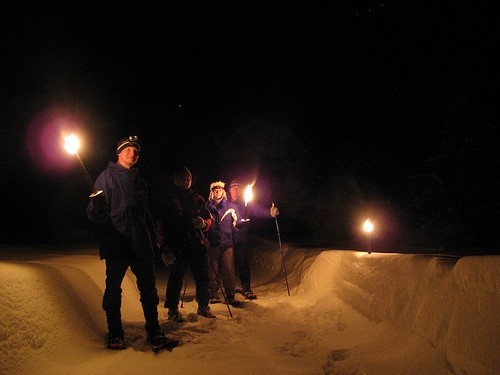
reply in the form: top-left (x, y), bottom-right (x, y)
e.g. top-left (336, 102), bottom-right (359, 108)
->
top-left (196, 303), bottom-right (216, 318)
top-left (210, 297), bottom-right (222, 303)
top-left (106, 329), bottom-right (126, 349)
top-left (224, 297), bottom-right (241, 306)
top-left (144, 332), bottom-right (179, 347)
top-left (240, 288), bottom-right (257, 299)
top-left (168, 306), bottom-right (184, 322)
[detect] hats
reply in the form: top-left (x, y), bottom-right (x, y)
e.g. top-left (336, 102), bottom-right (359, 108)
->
top-left (230, 179), bottom-right (243, 187)
top-left (116, 135), bottom-right (141, 154)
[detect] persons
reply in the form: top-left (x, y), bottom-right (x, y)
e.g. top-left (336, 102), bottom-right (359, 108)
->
top-left (156, 166), bottom-right (216, 323)
top-left (85, 134), bottom-right (179, 350)
top-left (204, 181), bottom-right (251, 306)
top-left (227, 179), bottom-right (279, 299)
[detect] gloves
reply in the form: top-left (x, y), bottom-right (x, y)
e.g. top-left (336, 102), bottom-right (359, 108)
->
top-left (161, 246), bottom-right (176, 266)
top-left (193, 216), bottom-right (206, 229)
